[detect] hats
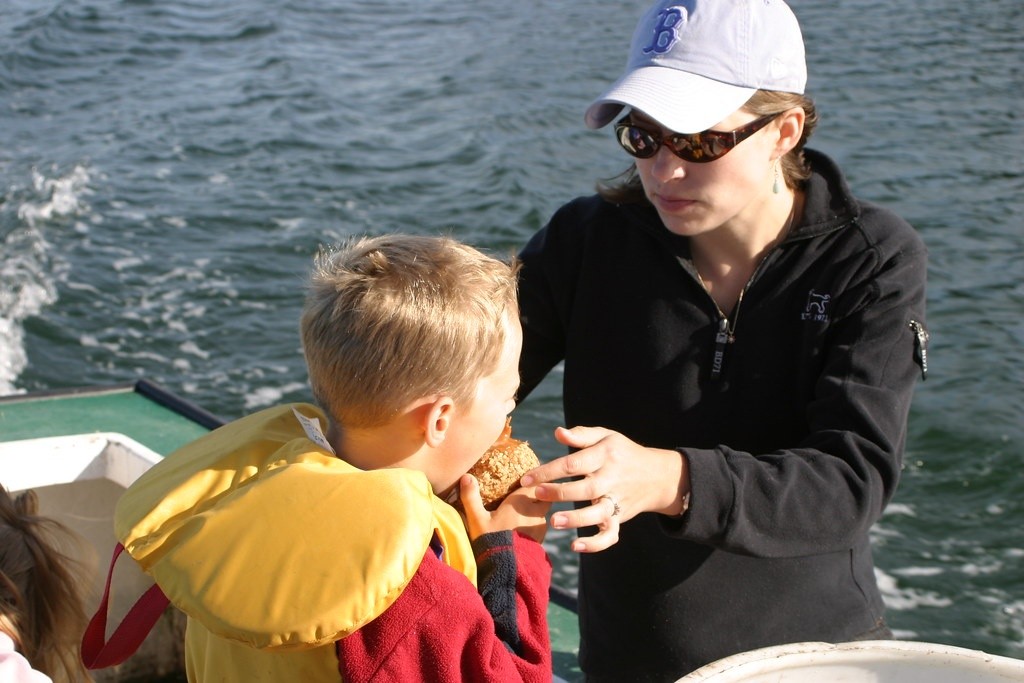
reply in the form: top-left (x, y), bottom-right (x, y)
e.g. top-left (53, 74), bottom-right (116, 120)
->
top-left (585, 0), bottom-right (807, 135)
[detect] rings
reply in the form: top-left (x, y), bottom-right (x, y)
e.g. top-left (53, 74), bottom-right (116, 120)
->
top-left (598, 493), bottom-right (621, 517)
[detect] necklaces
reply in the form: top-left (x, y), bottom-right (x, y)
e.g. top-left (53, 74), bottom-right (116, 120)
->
top-left (693, 264), bottom-right (744, 343)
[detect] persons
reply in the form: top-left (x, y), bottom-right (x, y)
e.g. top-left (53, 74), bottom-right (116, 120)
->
top-left (114, 228), bottom-right (554, 683)
top-left (0, 480), bottom-right (97, 683)
top-left (506, 0), bottom-right (929, 683)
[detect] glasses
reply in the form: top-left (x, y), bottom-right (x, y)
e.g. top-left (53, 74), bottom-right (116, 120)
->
top-left (613, 112), bottom-right (785, 164)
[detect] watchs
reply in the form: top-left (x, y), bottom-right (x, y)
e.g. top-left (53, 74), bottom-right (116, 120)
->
top-left (670, 493), bottom-right (692, 523)
